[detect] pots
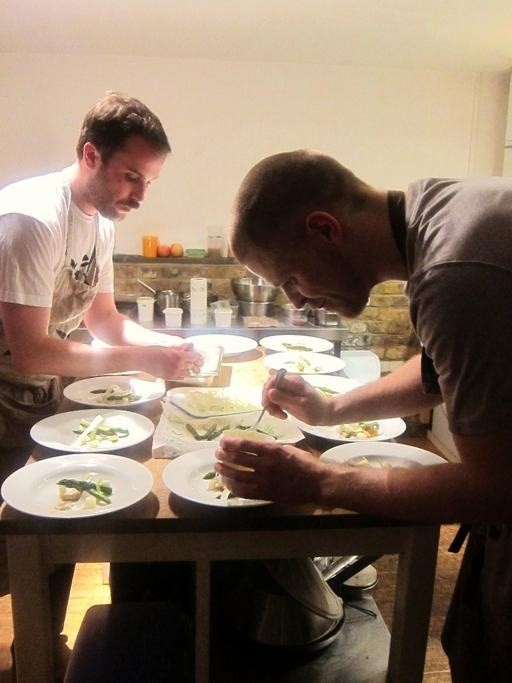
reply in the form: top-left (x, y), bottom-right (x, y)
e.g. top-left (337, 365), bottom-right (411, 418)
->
top-left (137, 278), bottom-right (181, 313)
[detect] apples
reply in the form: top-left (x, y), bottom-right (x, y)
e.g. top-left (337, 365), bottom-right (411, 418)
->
top-left (157, 245), bottom-right (170, 256)
top-left (170, 244), bottom-right (184, 257)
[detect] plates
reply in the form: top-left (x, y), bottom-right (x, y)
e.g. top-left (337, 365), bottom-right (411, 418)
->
top-left (318, 441), bottom-right (449, 472)
top-left (160, 441), bottom-right (280, 509)
top-left (29, 407), bottom-right (156, 452)
top-left (165, 334), bottom-right (407, 443)
top-left (62, 372), bottom-right (165, 408)
top-left (0, 453), bottom-right (156, 521)
top-left (185, 249), bottom-right (205, 259)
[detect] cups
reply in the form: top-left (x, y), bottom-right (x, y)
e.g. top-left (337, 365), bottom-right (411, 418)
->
top-left (209, 235), bottom-right (223, 258)
top-left (214, 308), bottom-right (232, 328)
top-left (162, 307), bottom-right (184, 328)
top-left (142, 236), bottom-right (157, 258)
top-left (136, 297), bottom-right (154, 321)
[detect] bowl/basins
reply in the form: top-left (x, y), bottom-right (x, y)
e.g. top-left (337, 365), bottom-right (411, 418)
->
top-left (230, 278), bottom-right (277, 318)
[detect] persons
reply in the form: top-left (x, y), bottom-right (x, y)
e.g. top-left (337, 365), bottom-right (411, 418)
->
top-left (215, 151), bottom-right (512, 683)
top-left (0, 95), bottom-right (204, 681)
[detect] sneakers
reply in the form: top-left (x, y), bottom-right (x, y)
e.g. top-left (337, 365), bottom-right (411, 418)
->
top-left (6, 630), bottom-right (76, 682)
